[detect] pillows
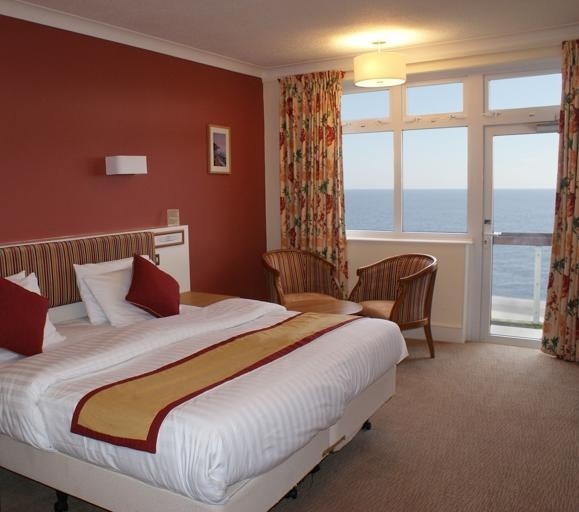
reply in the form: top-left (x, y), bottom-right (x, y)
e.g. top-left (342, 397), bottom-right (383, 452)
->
top-left (0, 268), bottom-right (62, 363)
top-left (75, 255), bottom-right (182, 326)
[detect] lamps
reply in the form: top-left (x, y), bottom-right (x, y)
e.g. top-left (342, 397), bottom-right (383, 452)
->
top-left (351, 41), bottom-right (408, 90)
top-left (105, 155), bottom-right (147, 175)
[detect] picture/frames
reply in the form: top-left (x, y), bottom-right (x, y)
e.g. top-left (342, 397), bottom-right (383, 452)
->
top-left (208, 124), bottom-right (234, 176)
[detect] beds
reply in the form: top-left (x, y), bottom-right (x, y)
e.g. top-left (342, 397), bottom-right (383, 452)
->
top-left (0, 233), bottom-right (399, 511)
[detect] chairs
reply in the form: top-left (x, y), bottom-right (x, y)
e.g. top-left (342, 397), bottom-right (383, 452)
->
top-left (264, 249), bottom-right (438, 359)
